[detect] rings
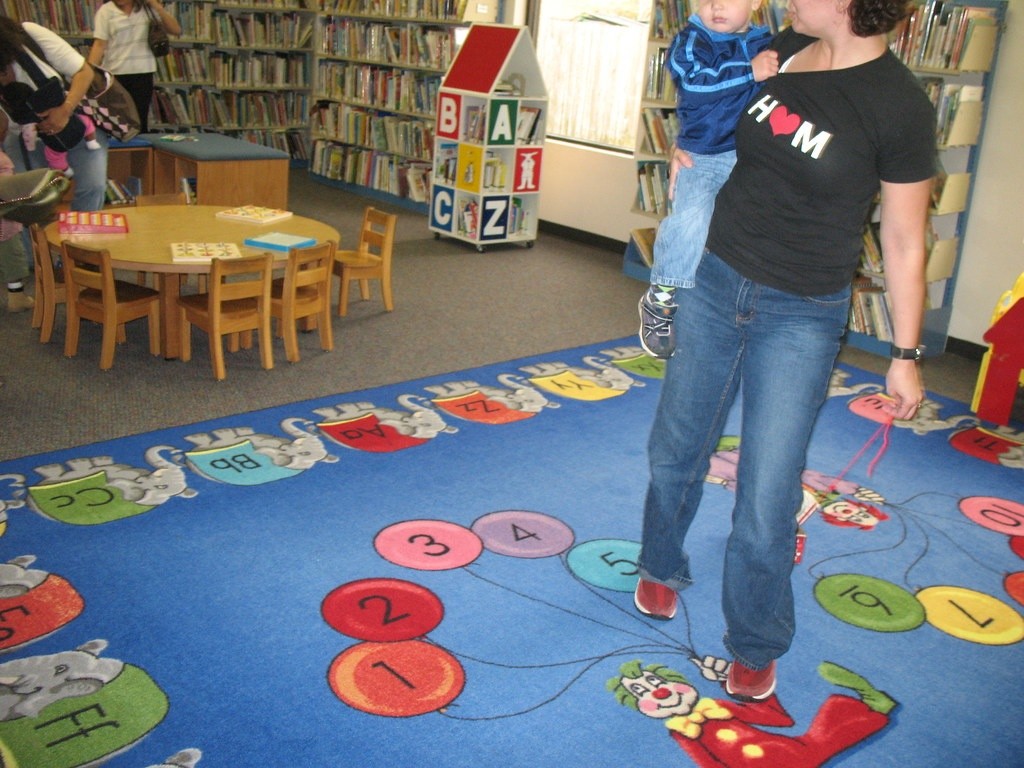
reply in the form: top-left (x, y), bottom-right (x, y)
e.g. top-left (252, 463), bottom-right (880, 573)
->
top-left (918, 403), bottom-right (922, 408)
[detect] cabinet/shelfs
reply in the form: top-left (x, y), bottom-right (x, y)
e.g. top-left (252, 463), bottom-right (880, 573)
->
top-left (308, 0), bottom-right (549, 250)
top-left (0, 0), bottom-right (310, 168)
top-left (136, 132), bottom-right (291, 209)
top-left (625, 0), bottom-right (1009, 362)
top-left (53, 134), bottom-right (154, 211)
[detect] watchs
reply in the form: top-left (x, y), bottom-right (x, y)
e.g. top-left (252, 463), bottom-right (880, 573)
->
top-left (890, 344), bottom-right (926, 363)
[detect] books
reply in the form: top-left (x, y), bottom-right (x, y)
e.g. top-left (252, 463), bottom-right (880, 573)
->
top-left (631, 0), bottom-right (995, 344)
top-left (148, 0), bottom-right (502, 202)
top-left (59, 210), bottom-right (129, 239)
top-left (170, 242), bottom-right (242, 263)
top-left (214, 206), bottom-right (293, 223)
top-left (0, 0), bottom-right (105, 59)
top-left (244, 232), bottom-right (317, 253)
top-left (435, 81), bottom-right (542, 239)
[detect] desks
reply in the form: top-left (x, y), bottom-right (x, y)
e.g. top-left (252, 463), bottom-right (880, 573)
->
top-left (44, 204), bottom-right (340, 360)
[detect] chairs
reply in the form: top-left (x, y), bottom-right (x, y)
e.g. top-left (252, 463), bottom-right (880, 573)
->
top-left (28, 193), bottom-right (336, 381)
top-left (333, 206), bottom-right (399, 317)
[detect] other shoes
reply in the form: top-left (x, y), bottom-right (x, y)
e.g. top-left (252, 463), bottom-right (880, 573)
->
top-left (52, 266), bottom-right (66, 282)
top-left (8, 292), bottom-right (36, 313)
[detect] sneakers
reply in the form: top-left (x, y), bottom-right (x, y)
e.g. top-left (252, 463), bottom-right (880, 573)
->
top-left (638, 283), bottom-right (679, 361)
top-left (726, 653), bottom-right (776, 701)
top-left (635, 574), bottom-right (676, 621)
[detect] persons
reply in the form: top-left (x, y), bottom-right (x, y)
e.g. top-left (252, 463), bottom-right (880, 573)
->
top-left (89, 0), bottom-right (182, 134)
top-left (633, 0), bottom-right (935, 701)
top-left (0, 17), bottom-right (110, 314)
top-left (638, 0), bottom-right (780, 359)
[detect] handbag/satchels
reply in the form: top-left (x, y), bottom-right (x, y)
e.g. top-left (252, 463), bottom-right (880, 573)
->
top-left (62, 60), bottom-right (141, 143)
top-left (143, 2), bottom-right (170, 57)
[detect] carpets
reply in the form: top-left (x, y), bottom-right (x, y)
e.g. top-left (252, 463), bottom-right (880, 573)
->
top-left (0, 334), bottom-right (1024, 768)
